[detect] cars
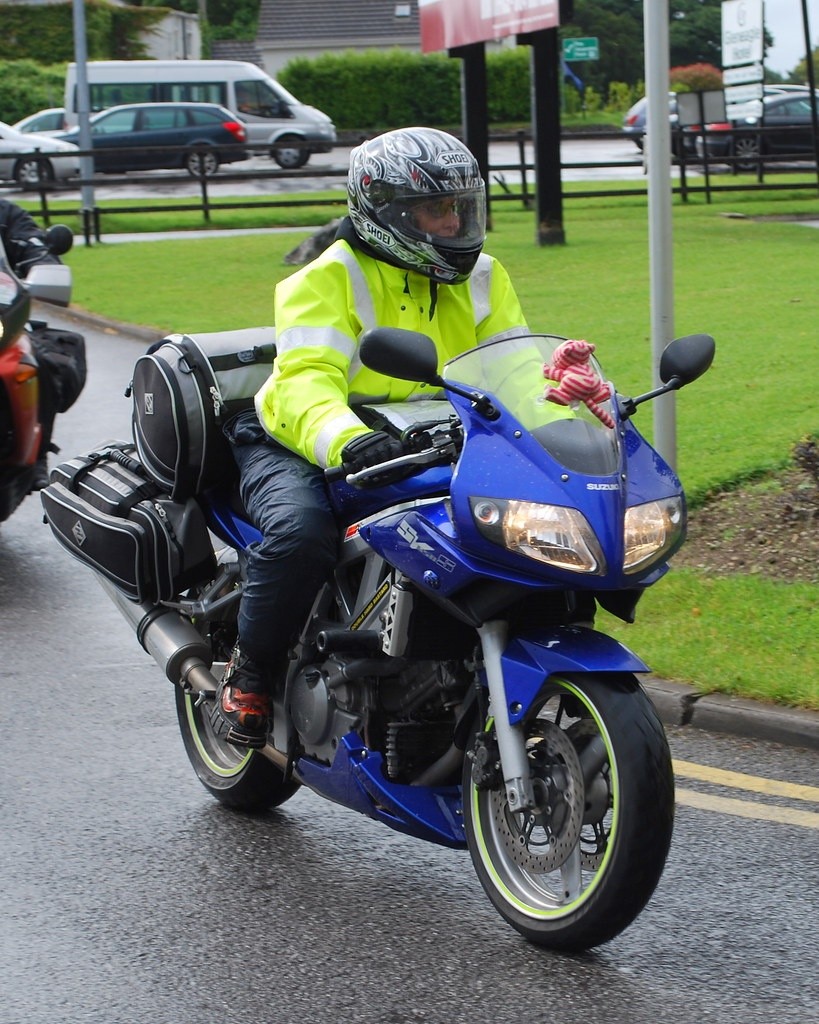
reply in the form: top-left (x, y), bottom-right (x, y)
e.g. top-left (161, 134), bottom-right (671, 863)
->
top-left (0, 121), bottom-right (82, 191)
top-left (11, 106), bottom-right (65, 136)
top-left (52, 101), bottom-right (254, 179)
top-left (620, 81), bottom-right (819, 172)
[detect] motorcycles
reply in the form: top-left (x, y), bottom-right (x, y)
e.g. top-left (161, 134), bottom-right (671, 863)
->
top-left (0, 219), bottom-right (74, 522)
top-left (36, 325), bottom-right (717, 954)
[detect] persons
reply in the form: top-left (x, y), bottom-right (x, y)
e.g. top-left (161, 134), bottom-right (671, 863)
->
top-left (0, 199), bottom-right (86, 491)
top-left (209, 127), bottom-right (577, 750)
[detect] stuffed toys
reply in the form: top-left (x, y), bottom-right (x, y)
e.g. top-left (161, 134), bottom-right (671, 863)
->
top-left (541, 340), bottom-right (617, 429)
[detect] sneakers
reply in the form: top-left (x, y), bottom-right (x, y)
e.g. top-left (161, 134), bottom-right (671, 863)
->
top-left (209, 639), bottom-right (275, 748)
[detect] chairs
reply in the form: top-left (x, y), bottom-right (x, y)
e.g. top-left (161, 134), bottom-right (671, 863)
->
top-left (110, 89), bottom-right (122, 105)
top-left (142, 115), bottom-right (150, 129)
top-left (150, 88), bottom-right (157, 102)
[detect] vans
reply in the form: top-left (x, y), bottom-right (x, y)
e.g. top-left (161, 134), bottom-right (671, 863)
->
top-left (62, 58), bottom-right (339, 173)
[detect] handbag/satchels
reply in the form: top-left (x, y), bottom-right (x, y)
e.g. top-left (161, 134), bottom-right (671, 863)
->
top-left (127, 324), bottom-right (276, 503)
top-left (24, 318), bottom-right (87, 413)
top-left (38, 438), bottom-right (218, 607)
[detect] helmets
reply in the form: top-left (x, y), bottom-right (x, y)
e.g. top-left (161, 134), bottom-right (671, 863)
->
top-left (345, 128), bottom-right (486, 285)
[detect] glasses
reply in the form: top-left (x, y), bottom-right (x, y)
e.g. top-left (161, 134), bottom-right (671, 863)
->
top-left (422, 198), bottom-right (468, 217)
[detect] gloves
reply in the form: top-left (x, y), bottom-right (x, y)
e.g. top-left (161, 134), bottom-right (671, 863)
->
top-left (340, 430), bottom-right (402, 482)
